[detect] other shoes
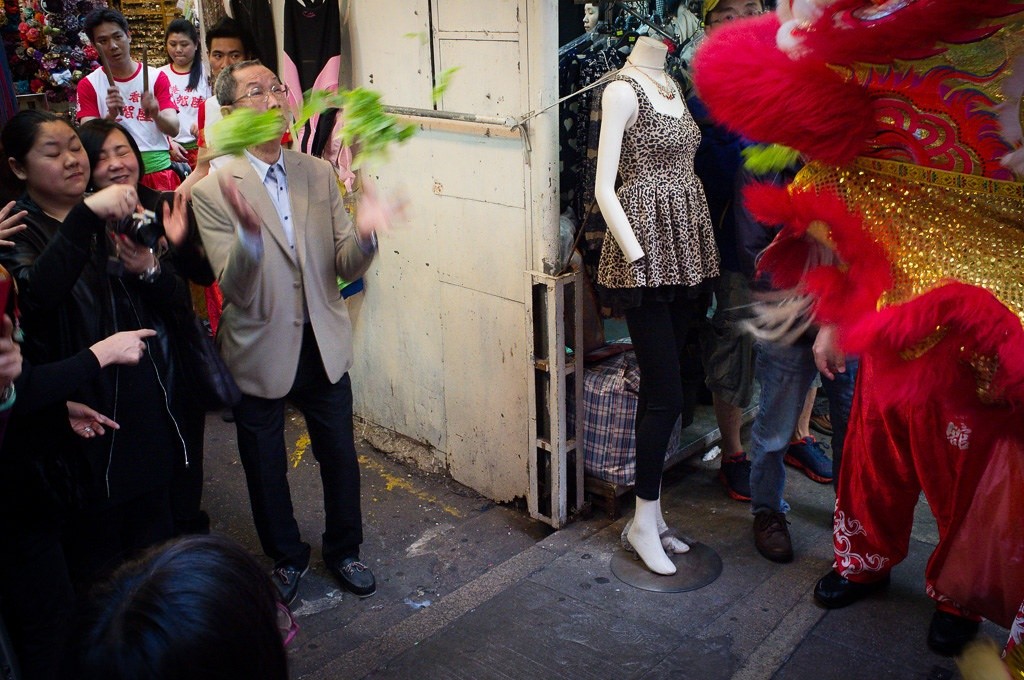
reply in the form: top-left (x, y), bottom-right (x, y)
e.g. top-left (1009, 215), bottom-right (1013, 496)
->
top-left (810, 414), bottom-right (831, 433)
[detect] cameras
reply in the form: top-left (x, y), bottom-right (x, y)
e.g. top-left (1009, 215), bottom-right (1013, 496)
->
top-left (105, 207), bottom-right (165, 247)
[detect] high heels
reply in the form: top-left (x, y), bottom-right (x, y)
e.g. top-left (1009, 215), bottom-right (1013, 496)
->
top-left (928, 611), bottom-right (980, 656)
top-left (814, 569), bottom-right (891, 609)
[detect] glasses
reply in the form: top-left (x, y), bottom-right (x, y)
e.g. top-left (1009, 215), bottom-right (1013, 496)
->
top-left (708, 10), bottom-right (770, 25)
top-left (233, 84), bottom-right (289, 102)
top-left (273, 601), bottom-right (301, 644)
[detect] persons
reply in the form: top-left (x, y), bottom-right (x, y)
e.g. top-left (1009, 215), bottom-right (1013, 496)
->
top-left (0, 200), bottom-right (159, 439)
top-left (189, 58), bottom-right (383, 607)
top-left (593, 36), bottom-right (722, 575)
top-left (156, 18), bottom-right (212, 182)
top-left (174, 15), bottom-right (293, 204)
top-left (75, 7), bottom-right (181, 192)
top-left (583, 0), bottom-right (600, 36)
top-left (76, 534), bottom-right (300, 680)
top-left (0, 108), bottom-right (217, 537)
top-left (684, 0), bottom-right (1024, 679)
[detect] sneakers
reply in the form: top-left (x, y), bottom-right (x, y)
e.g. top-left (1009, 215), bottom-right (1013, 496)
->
top-left (719, 451), bottom-right (751, 500)
top-left (784, 436), bottom-right (833, 482)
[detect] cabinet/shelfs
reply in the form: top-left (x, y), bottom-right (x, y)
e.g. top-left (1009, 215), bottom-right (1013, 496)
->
top-left (533, 309), bottom-right (760, 523)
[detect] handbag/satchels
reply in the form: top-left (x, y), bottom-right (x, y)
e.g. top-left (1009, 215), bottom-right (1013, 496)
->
top-left (567, 351), bottom-right (684, 486)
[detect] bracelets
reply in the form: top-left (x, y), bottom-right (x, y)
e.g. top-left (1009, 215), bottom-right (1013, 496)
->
top-left (352, 228), bottom-right (379, 253)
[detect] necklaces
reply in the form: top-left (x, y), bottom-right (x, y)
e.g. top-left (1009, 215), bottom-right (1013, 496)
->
top-left (625, 58), bottom-right (677, 101)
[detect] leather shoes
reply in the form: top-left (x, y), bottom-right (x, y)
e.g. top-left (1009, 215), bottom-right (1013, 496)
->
top-left (269, 566), bottom-right (308, 605)
top-left (754, 512), bottom-right (794, 564)
top-left (332, 556), bottom-right (376, 598)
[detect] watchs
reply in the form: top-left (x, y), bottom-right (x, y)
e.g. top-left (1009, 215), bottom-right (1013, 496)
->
top-left (140, 257), bottom-right (161, 283)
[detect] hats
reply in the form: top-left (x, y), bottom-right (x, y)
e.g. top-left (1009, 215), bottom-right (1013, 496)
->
top-left (702, 0), bottom-right (720, 23)
